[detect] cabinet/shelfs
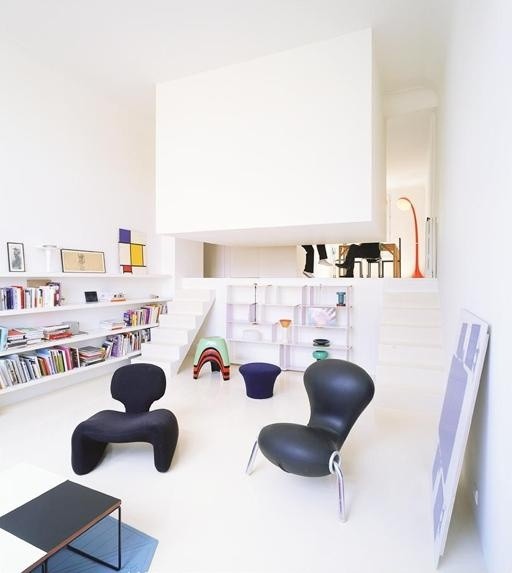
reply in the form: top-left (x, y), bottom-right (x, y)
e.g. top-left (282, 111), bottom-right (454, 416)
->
top-left (221, 282), bottom-right (352, 371)
top-left (0, 270), bottom-right (173, 394)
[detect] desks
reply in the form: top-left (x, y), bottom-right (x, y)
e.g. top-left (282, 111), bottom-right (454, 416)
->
top-left (339, 243), bottom-right (399, 278)
top-left (0, 478), bottom-right (123, 573)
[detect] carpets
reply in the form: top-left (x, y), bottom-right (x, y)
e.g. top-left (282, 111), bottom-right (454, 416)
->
top-left (31, 515), bottom-right (160, 573)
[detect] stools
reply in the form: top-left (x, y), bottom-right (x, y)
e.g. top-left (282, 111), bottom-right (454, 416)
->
top-left (190, 335), bottom-right (282, 400)
top-left (353, 256), bottom-right (393, 277)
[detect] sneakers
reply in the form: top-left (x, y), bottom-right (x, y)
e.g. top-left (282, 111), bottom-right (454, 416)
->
top-left (304, 260), bottom-right (353, 278)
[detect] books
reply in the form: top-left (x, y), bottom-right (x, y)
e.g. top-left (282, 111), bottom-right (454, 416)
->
top-left (0, 285), bottom-right (167, 387)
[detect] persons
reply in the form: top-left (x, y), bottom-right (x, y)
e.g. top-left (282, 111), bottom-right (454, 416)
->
top-left (335, 243), bottom-right (380, 276)
top-left (302, 244), bottom-right (333, 279)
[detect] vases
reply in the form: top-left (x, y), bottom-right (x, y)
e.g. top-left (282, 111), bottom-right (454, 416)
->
top-left (312, 350), bottom-right (328, 361)
top-left (335, 290), bottom-right (346, 306)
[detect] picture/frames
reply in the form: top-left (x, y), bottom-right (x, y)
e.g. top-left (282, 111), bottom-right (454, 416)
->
top-left (7, 241), bottom-right (26, 272)
top-left (60, 247), bottom-right (106, 273)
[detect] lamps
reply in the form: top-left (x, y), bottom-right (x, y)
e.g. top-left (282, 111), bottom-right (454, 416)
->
top-left (395, 195), bottom-right (424, 278)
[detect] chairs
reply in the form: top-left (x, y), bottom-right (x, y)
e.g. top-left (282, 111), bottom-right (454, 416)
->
top-left (244, 358), bottom-right (376, 521)
top-left (72, 358), bottom-right (182, 476)
